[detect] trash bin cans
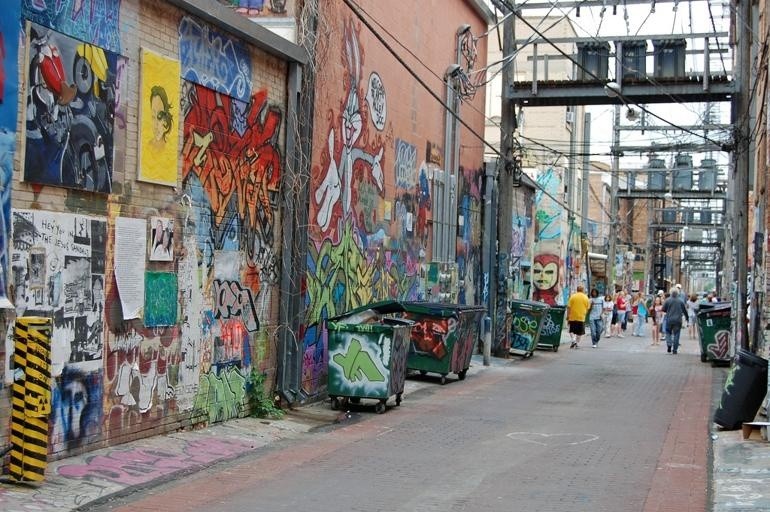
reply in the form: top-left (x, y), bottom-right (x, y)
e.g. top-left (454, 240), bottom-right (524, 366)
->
top-left (714, 349), bottom-right (768, 429)
top-left (693, 302), bottom-right (731, 367)
top-left (506, 299), bottom-right (567, 358)
top-left (327, 300), bottom-right (489, 414)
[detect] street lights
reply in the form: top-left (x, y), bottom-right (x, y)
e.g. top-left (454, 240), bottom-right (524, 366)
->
top-left (717, 270), bottom-right (723, 297)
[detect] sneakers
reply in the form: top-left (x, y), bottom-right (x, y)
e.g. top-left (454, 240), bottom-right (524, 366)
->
top-left (667, 346), bottom-right (677, 354)
top-left (570, 341), bottom-right (580, 348)
top-left (592, 344), bottom-right (596, 347)
top-left (605, 333), bottom-right (624, 338)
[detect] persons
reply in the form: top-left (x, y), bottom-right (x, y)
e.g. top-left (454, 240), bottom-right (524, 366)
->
top-left (152, 220), bottom-right (173, 260)
top-left (150, 85), bottom-right (174, 147)
top-left (567, 284), bottom-right (721, 355)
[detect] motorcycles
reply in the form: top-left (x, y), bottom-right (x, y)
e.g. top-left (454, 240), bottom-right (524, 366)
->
top-left (29, 22), bottom-right (117, 193)
